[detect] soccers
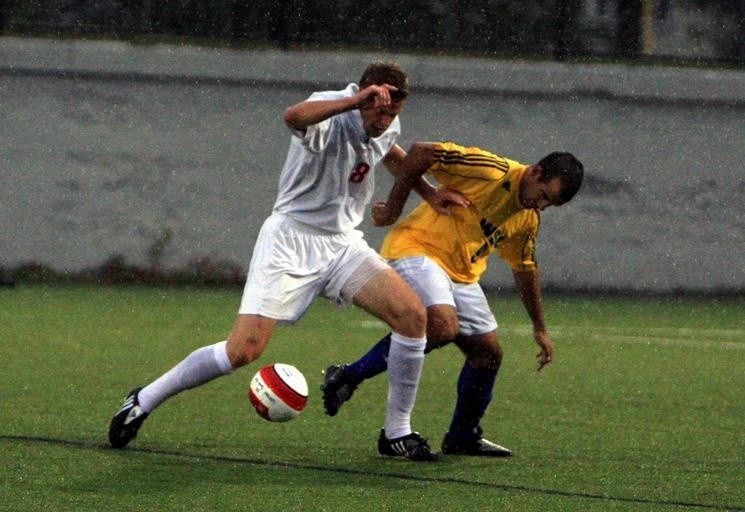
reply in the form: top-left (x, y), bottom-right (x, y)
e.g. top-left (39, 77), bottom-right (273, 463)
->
top-left (248, 363), bottom-right (309, 422)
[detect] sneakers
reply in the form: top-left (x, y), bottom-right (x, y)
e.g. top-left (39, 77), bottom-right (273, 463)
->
top-left (442, 428), bottom-right (512, 457)
top-left (378, 427), bottom-right (439, 461)
top-left (109, 387), bottom-right (149, 449)
top-left (321, 365), bottom-right (358, 417)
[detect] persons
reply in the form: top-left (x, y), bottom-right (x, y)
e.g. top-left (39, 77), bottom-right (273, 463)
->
top-left (104, 61), bottom-right (442, 467)
top-left (322, 138), bottom-right (583, 460)
